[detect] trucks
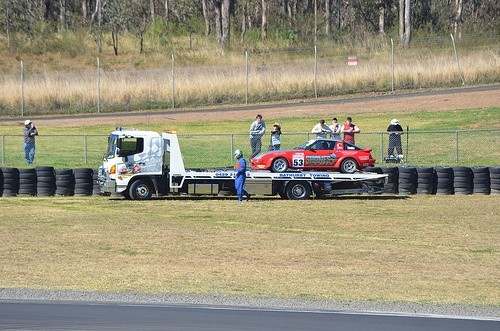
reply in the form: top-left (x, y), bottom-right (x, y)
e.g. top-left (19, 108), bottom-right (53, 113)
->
top-left (97, 129), bottom-right (391, 200)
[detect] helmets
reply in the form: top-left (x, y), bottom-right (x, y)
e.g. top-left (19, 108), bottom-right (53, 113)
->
top-left (235, 150), bottom-right (243, 159)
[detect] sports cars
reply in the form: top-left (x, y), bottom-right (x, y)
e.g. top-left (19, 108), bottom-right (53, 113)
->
top-left (248, 137), bottom-right (375, 174)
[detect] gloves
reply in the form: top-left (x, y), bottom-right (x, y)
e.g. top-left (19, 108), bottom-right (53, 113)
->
top-left (233, 172), bottom-right (237, 176)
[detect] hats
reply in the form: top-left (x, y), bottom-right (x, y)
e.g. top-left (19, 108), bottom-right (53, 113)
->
top-left (391, 118), bottom-right (399, 125)
top-left (273, 122), bottom-right (281, 128)
top-left (25, 120), bottom-right (32, 125)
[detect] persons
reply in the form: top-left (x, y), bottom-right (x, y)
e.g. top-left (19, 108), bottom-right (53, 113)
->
top-left (330, 118), bottom-right (341, 141)
top-left (269, 123), bottom-right (282, 150)
top-left (234, 150), bottom-right (250, 202)
top-left (23, 120), bottom-right (38, 164)
top-left (250, 114), bottom-right (265, 159)
top-left (342, 118), bottom-right (360, 146)
top-left (312, 119), bottom-right (332, 140)
top-left (386, 118), bottom-right (404, 159)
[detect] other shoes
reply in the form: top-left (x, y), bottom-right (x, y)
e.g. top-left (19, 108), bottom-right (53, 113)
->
top-left (247, 194), bottom-right (251, 201)
